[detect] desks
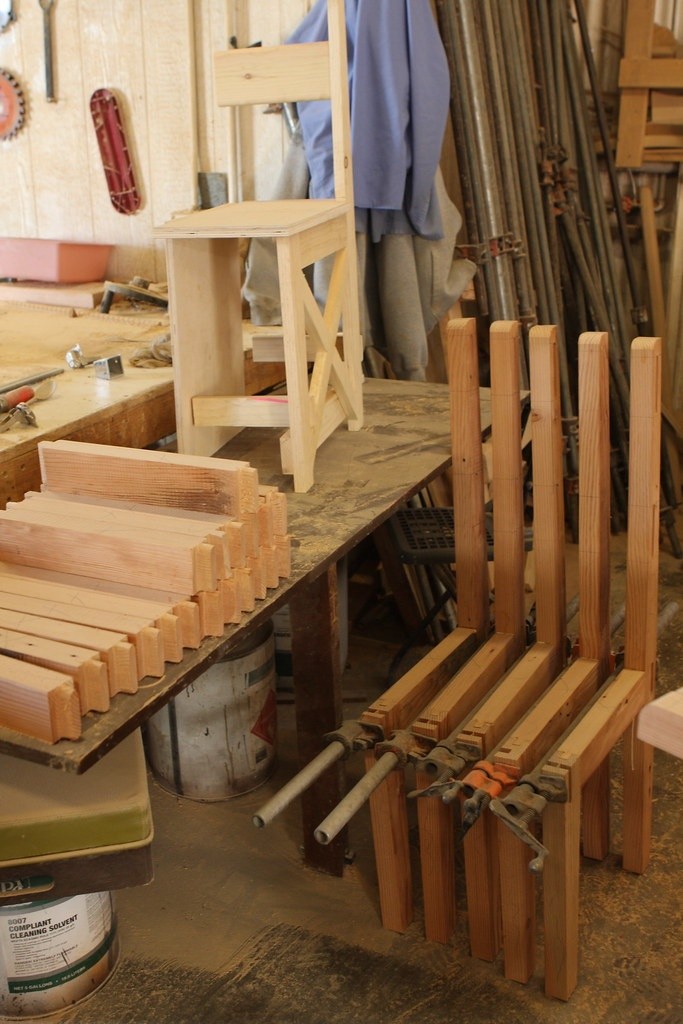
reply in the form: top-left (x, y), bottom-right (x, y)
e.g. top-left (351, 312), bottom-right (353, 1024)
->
top-left (0, 360), bottom-right (313, 511)
top-left (0, 374), bottom-right (532, 875)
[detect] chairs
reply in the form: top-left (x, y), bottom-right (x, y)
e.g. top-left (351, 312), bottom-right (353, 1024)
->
top-left (153, 0), bottom-right (364, 494)
top-left (352, 345), bottom-right (532, 686)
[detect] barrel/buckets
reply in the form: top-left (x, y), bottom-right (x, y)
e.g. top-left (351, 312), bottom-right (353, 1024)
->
top-left (149, 618), bottom-right (278, 802)
top-left (0, 890), bottom-right (121, 1020)
top-left (273, 605), bottom-right (294, 690)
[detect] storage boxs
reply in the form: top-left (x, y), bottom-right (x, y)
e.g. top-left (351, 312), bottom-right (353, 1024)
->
top-left (1, 727), bottom-right (153, 907)
